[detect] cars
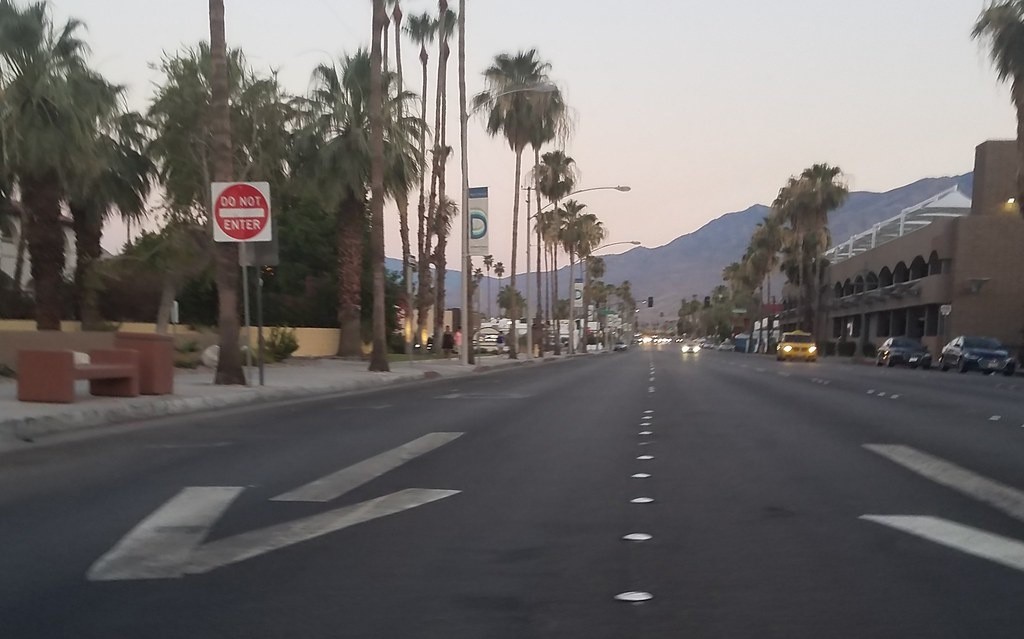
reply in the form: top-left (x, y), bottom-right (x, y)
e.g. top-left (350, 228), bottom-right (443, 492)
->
top-left (776, 330), bottom-right (817, 362)
top-left (939, 336), bottom-right (1016, 377)
top-left (874, 336), bottom-right (932, 370)
top-left (614, 333), bottom-right (735, 354)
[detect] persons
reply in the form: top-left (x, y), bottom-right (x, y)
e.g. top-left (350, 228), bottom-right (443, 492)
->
top-left (496, 331), bottom-right (505, 357)
top-left (442, 325), bottom-right (462, 361)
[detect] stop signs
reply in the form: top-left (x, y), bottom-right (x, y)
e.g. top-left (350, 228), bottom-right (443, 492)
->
top-left (214, 183), bottom-right (269, 240)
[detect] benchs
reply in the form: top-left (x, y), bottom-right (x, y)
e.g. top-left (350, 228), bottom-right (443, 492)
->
top-left (17, 349), bottom-right (139, 404)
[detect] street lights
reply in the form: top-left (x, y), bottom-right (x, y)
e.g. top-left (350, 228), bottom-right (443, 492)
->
top-left (527, 186), bottom-right (631, 358)
top-left (567, 241), bottom-right (641, 354)
top-left (462, 85), bottom-right (556, 362)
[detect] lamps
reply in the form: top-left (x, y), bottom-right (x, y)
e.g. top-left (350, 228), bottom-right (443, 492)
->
top-left (1007, 197), bottom-right (1016, 203)
